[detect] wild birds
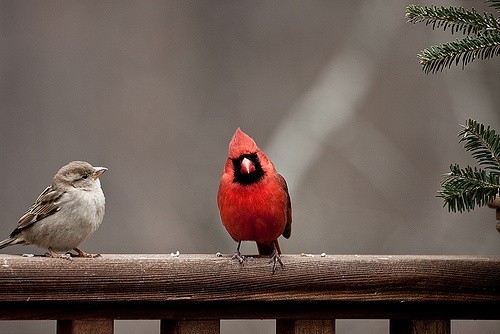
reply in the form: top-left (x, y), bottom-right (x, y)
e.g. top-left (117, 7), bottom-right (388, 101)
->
top-left (0, 160), bottom-right (109, 260)
top-left (216, 126), bottom-right (292, 274)
